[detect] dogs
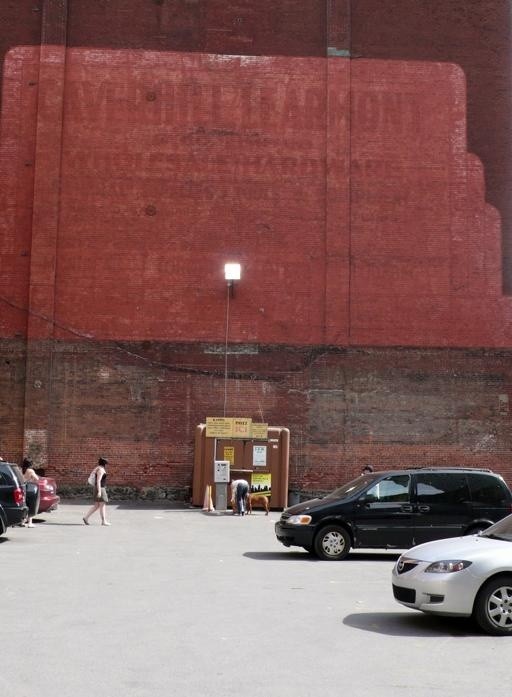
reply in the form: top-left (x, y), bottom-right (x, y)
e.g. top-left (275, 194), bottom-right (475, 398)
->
top-left (245, 491), bottom-right (269, 515)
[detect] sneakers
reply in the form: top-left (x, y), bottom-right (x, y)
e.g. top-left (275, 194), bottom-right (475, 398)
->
top-left (238, 511), bottom-right (245, 516)
top-left (83, 517), bottom-right (90, 525)
top-left (101, 521), bottom-right (113, 527)
top-left (20, 523), bottom-right (36, 527)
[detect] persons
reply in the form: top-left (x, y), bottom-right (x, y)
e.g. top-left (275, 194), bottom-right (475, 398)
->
top-left (83, 456), bottom-right (111, 525)
top-left (19, 456), bottom-right (40, 525)
top-left (228, 477), bottom-right (249, 516)
top-left (360, 464), bottom-right (381, 500)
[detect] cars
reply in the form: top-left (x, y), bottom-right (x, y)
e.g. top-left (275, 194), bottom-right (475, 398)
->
top-left (0, 460), bottom-right (60, 536)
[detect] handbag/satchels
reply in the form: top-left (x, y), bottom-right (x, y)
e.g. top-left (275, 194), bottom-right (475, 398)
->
top-left (25, 481), bottom-right (39, 497)
top-left (87, 467), bottom-right (100, 487)
